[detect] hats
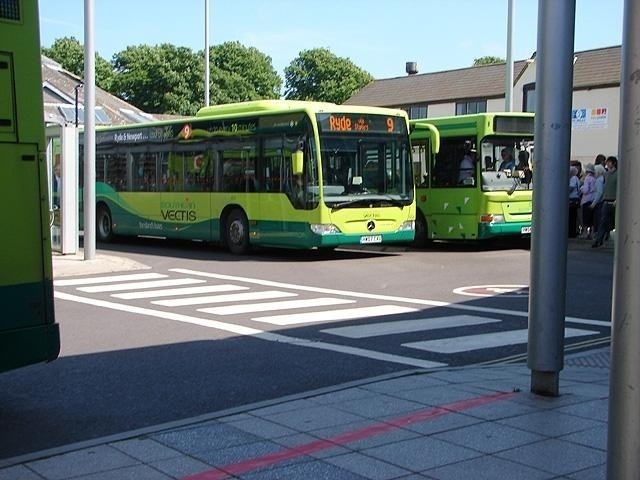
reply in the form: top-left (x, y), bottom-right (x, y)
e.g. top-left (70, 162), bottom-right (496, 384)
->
top-left (583, 163), bottom-right (595, 174)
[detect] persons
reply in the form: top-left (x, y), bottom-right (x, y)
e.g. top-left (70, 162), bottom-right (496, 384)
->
top-left (456, 145), bottom-right (530, 184)
top-left (567, 153), bottom-right (620, 247)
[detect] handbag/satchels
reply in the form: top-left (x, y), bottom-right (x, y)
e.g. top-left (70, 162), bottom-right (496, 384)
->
top-left (570, 197), bottom-right (581, 209)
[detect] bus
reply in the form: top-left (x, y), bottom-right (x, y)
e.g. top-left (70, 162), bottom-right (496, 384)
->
top-left (409, 111), bottom-right (535, 248)
top-left (1, 2), bottom-right (65, 378)
top-left (79, 98), bottom-right (445, 256)
